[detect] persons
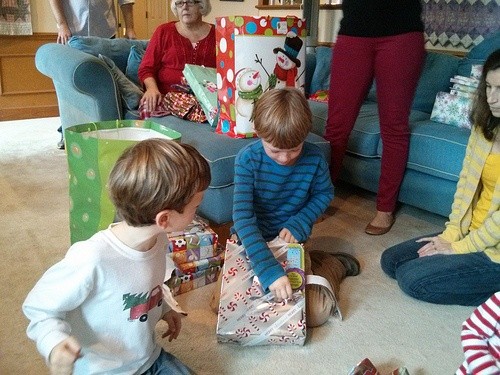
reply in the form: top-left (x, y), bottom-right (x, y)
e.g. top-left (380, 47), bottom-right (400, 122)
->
top-left (454, 291), bottom-right (500, 375)
top-left (326, 0), bottom-right (425, 236)
top-left (138, 0), bottom-right (217, 123)
top-left (22, 136), bottom-right (212, 375)
top-left (49, 0), bottom-right (137, 149)
top-left (379, 48), bottom-right (500, 304)
top-left (229, 88), bottom-right (361, 329)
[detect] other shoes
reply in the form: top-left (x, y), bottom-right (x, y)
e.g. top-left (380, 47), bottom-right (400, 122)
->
top-left (57, 138), bottom-right (65, 149)
top-left (364, 216), bottom-right (395, 236)
top-left (329, 251), bottom-right (361, 276)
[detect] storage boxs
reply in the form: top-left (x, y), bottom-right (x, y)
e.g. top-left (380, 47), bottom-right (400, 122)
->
top-left (430, 91), bottom-right (474, 130)
top-left (165, 215), bottom-right (220, 296)
top-left (215, 240), bottom-right (305, 345)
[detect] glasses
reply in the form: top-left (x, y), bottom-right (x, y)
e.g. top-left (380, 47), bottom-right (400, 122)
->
top-left (175, 0), bottom-right (202, 7)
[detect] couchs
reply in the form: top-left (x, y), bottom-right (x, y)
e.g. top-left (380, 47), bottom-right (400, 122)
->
top-left (33, 28), bottom-right (500, 224)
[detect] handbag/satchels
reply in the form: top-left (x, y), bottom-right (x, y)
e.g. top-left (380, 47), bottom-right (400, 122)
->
top-left (215, 16), bottom-right (307, 138)
top-left (65, 120), bottom-right (183, 247)
